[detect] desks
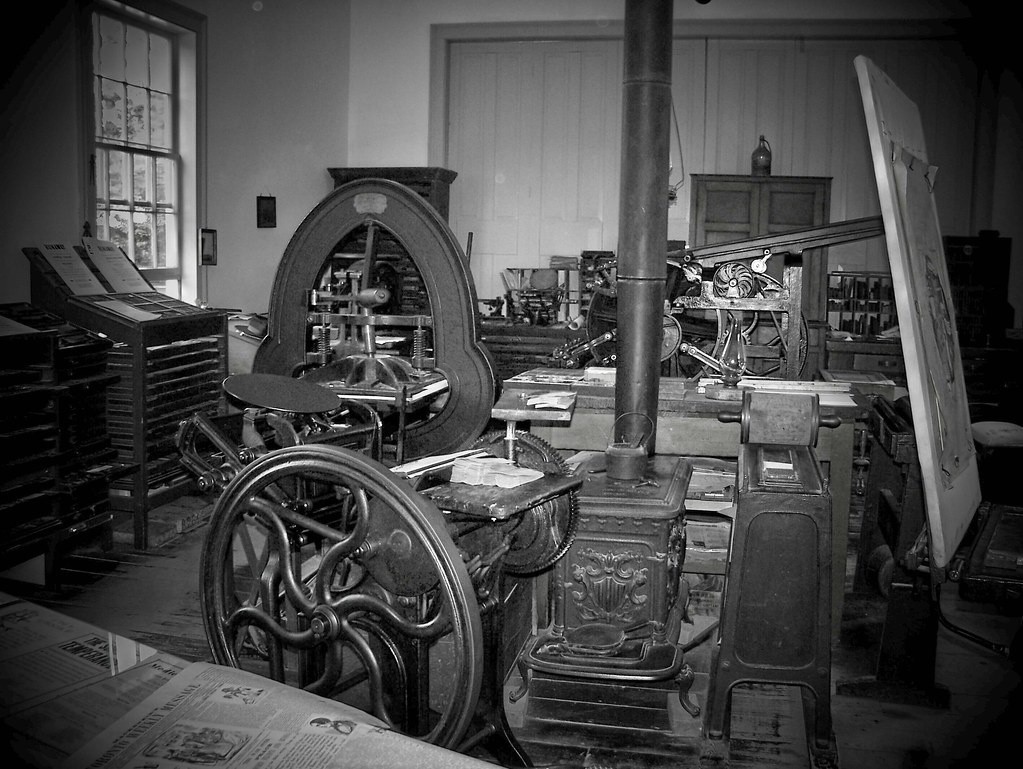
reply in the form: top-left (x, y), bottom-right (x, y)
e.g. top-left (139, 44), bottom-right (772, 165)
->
top-left (502, 366), bottom-right (858, 653)
top-left (853, 415), bottom-right (1023, 694)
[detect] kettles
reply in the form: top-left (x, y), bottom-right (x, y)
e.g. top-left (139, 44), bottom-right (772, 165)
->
top-left (604, 413), bottom-right (655, 480)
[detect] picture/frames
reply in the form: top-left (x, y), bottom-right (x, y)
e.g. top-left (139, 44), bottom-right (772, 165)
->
top-left (257, 195), bottom-right (276, 227)
top-left (198, 228), bottom-right (217, 266)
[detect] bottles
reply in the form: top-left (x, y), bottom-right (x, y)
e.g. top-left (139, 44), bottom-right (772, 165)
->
top-left (751, 136), bottom-right (772, 176)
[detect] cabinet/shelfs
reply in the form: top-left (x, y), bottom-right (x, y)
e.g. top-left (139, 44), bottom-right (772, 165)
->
top-left (825, 270), bottom-right (899, 335)
top-left (825, 340), bottom-right (1023, 426)
top-left (689, 172), bottom-right (833, 380)
